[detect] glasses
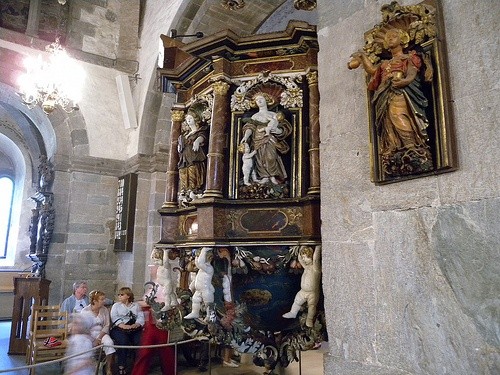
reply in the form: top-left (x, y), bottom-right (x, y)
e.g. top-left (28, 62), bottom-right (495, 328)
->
top-left (91, 290), bottom-right (101, 298)
top-left (117, 294), bottom-right (126, 297)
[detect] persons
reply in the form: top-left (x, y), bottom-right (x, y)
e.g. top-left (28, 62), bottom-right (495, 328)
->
top-left (56, 280), bottom-right (177, 375)
top-left (184, 249), bottom-right (215, 323)
top-left (349, 30), bottom-right (426, 157)
top-left (152, 247), bottom-right (182, 314)
top-left (281, 247), bottom-right (324, 328)
top-left (258, 113), bottom-right (284, 136)
top-left (240, 93), bottom-right (286, 184)
top-left (236, 142), bottom-right (258, 187)
top-left (178, 110), bottom-right (206, 200)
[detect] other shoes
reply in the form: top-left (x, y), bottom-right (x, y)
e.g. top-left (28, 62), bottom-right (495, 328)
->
top-left (118, 365), bottom-right (126, 375)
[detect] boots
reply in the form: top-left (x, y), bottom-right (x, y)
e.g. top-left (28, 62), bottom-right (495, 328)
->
top-left (105, 353), bottom-right (113, 375)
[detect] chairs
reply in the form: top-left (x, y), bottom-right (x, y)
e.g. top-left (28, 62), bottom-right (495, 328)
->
top-left (25, 304), bottom-right (63, 364)
top-left (28, 310), bottom-right (69, 375)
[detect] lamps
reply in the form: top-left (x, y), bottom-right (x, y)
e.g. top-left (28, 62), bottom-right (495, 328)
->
top-left (11, 1), bottom-right (87, 116)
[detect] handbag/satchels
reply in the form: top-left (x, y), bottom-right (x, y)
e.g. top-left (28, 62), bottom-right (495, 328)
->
top-left (116, 317), bottom-right (144, 335)
